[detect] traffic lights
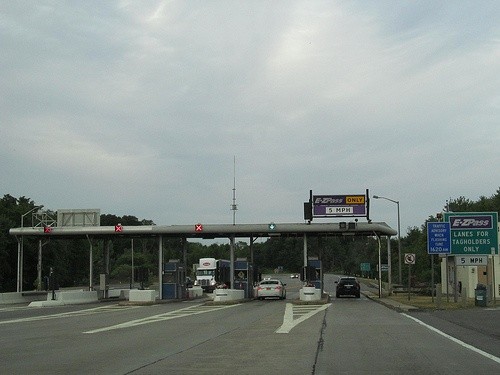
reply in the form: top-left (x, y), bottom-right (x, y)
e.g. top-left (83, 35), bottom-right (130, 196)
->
top-left (339, 221), bottom-right (346, 229)
top-left (115, 225), bottom-right (122, 232)
top-left (194, 225), bottom-right (203, 232)
top-left (269, 224), bottom-right (276, 230)
top-left (44, 226), bottom-right (51, 233)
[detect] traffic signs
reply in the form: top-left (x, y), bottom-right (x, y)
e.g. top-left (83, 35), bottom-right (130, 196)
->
top-left (443, 211), bottom-right (499, 256)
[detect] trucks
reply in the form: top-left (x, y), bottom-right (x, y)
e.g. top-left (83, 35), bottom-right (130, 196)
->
top-left (194, 257), bottom-right (231, 292)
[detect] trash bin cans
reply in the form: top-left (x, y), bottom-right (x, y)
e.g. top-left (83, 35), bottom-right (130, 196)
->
top-left (474, 286), bottom-right (486, 307)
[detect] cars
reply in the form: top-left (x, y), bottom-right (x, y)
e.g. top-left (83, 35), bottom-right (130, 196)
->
top-left (289, 274), bottom-right (299, 279)
top-left (257, 280), bottom-right (287, 300)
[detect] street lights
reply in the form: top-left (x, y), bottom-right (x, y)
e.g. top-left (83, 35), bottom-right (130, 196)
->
top-left (20, 204), bottom-right (44, 292)
top-left (373, 195), bottom-right (402, 285)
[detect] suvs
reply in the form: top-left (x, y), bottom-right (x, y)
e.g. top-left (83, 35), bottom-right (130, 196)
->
top-left (334, 277), bottom-right (361, 298)
